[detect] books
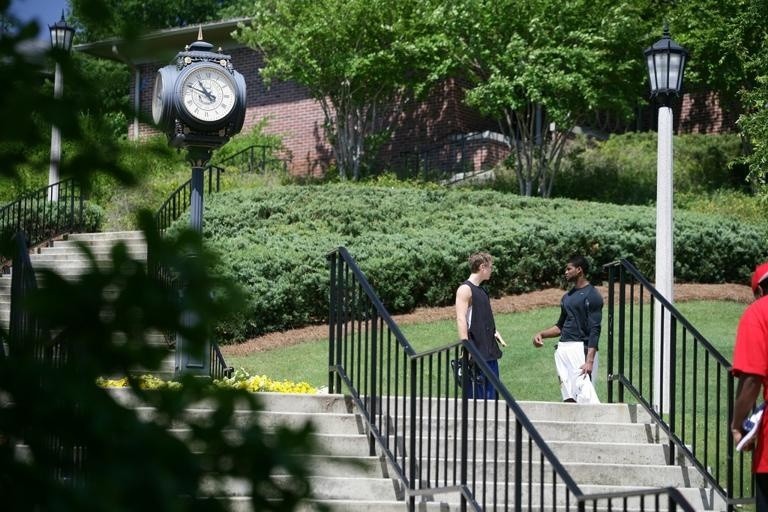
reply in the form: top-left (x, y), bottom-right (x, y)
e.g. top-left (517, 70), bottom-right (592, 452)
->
top-left (735, 401), bottom-right (765, 451)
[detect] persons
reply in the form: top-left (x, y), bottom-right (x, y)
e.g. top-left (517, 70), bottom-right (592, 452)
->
top-left (455, 248), bottom-right (507, 399)
top-left (532, 253), bottom-right (603, 405)
top-left (730, 260), bottom-right (768, 511)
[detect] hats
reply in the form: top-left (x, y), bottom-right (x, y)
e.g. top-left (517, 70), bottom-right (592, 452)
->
top-left (750, 262), bottom-right (768, 295)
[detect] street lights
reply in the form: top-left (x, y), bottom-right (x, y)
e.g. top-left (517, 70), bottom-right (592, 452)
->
top-left (46, 8), bottom-right (76, 204)
top-left (644, 21), bottom-right (680, 415)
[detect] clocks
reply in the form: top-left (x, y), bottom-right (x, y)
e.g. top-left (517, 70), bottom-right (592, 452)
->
top-left (150, 65), bottom-right (176, 134)
top-left (172, 61), bottom-right (240, 133)
